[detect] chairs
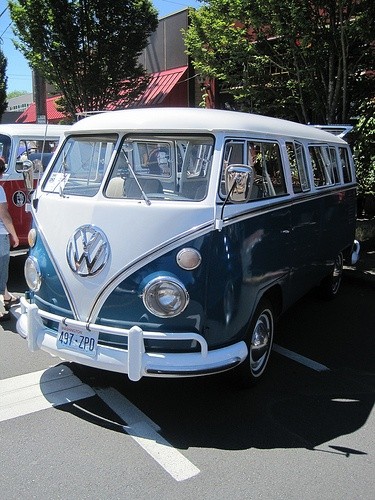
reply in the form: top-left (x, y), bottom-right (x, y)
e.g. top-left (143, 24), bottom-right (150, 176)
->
top-left (26, 152), bottom-right (55, 172)
top-left (123, 176), bottom-right (305, 203)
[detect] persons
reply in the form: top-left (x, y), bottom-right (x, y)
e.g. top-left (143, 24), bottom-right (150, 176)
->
top-left (0, 156), bottom-right (21, 321)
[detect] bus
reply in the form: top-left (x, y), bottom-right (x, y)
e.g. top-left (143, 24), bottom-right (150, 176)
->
top-left (0, 123), bottom-right (71, 253)
top-left (15, 103), bottom-right (360, 383)
